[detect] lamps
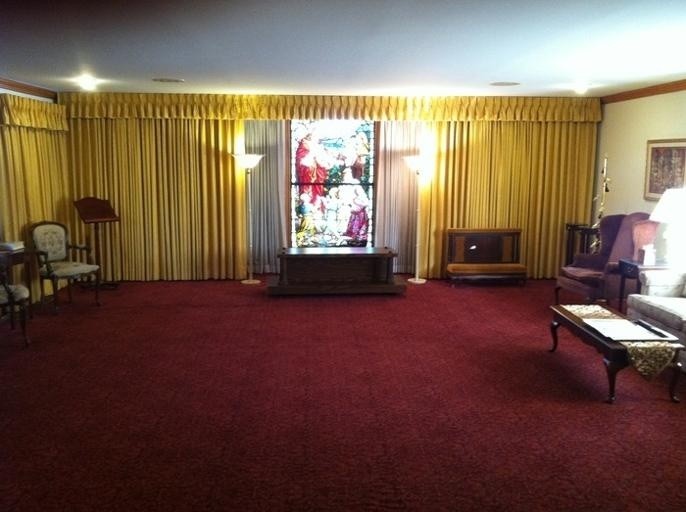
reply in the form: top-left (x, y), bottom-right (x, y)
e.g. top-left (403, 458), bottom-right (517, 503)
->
top-left (229, 152), bottom-right (266, 286)
top-left (401, 155), bottom-right (434, 285)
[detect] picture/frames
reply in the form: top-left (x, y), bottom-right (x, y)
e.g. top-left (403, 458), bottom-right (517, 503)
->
top-left (643, 137), bottom-right (686, 201)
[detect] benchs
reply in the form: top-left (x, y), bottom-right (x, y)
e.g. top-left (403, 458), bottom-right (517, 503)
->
top-left (446, 228), bottom-right (528, 287)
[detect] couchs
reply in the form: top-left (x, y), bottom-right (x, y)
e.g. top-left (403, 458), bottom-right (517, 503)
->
top-left (625, 270), bottom-right (686, 334)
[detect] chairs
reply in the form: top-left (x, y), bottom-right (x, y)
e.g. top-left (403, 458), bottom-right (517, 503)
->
top-left (0, 277), bottom-right (32, 346)
top-left (29, 220), bottom-right (103, 313)
top-left (554, 212), bottom-right (663, 306)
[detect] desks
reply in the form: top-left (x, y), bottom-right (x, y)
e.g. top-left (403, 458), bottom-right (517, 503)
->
top-left (548, 302), bottom-right (686, 405)
top-left (267, 245), bottom-right (406, 297)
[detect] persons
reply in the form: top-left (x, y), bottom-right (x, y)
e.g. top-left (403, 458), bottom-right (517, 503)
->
top-left (292, 122), bottom-right (370, 244)
